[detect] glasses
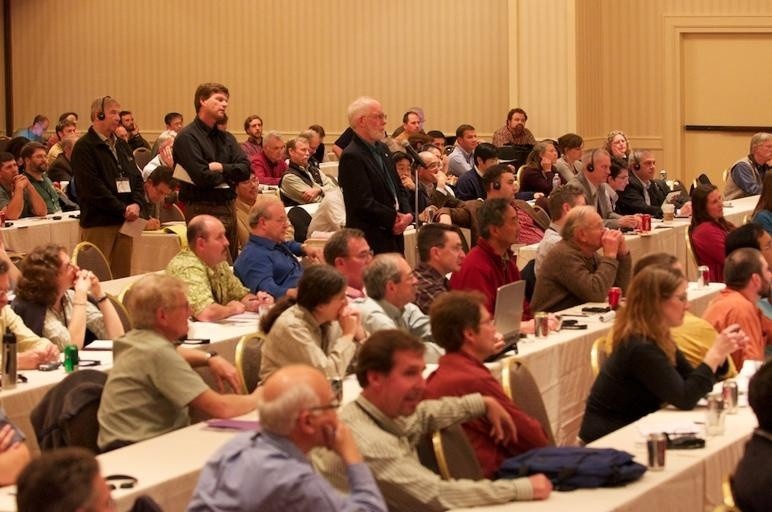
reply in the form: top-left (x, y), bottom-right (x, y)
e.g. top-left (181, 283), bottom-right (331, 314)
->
top-left (617, 176), bottom-right (629, 181)
top-left (480, 317), bottom-right (495, 327)
top-left (350, 249), bottom-right (374, 259)
top-left (309, 399), bottom-right (340, 412)
top-left (59, 259), bottom-right (73, 274)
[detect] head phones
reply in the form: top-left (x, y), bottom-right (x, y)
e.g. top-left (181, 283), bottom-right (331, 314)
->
top-left (96, 95), bottom-right (110, 120)
top-left (630, 151), bottom-right (642, 172)
top-left (584, 147), bottom-right (597, 173)
top-left (485, 164), bottom-right (503, 191)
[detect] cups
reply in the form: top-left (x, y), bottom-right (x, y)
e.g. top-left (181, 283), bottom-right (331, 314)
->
top-left (60, 181), bottom-right (69, 193)
top-left (663, 204), bottom-right (675, 225)
top-left (327, 151), bottom-right (335, 162)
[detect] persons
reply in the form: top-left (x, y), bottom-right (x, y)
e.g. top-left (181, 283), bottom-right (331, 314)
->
top-left (418, 291), bottom-right (556, 481)
top-left (0, 82), bottom-right (771, 382)
top-left (0, 407), bottom-right (30, 485)
top-left (573, 262), bottom-right (751, 448)
top-left (14, 448), bottom-right (120, 512)
top-left (186, 362), bottom-right (388, 511)
top-left (97, 271), bottom-right (268, 451)
top-left (729, 357), bottom-right (772, 511)
top-left (307, 329), bottom-right (553, 511)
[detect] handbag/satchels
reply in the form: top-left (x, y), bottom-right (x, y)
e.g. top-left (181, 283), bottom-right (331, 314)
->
top-left (494, 446), bottom-right (647, 491)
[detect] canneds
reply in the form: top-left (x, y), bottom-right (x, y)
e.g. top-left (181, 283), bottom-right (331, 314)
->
top-left (327, 376), bottom-right (343, 403)
top-left (647, 433), bottom-right (666, 470)
top-left (64, 345), bottom-right (77, 374)
top-left (708, 392), bottom-right (724, 427)
top-left (642, 214), bottom-right (651, 231)
top-left (723, 382), bottom-right (739, 415)
top-left (534, 311), bottom-right (548, 337)
top-left (608, 287), bottom-right (622, 310)
top-left (697, 266), bottom-right (710, 285)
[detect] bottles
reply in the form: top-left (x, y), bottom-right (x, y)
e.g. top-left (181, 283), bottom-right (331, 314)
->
top-left (2, 326), bottom-right (16, 390)
top-left (707, 397), bottom-right (725, 436)
top-left (660, 168), bottom-right (667, 185)
top-left (552, 173), bottom-right (561, 191)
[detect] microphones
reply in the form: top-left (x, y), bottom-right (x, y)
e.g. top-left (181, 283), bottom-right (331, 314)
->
top-left (397, 136), bottom-right (430, 171)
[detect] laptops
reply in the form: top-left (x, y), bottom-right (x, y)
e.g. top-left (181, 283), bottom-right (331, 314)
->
top-left (488, 280), bottom-right (526, 347)
